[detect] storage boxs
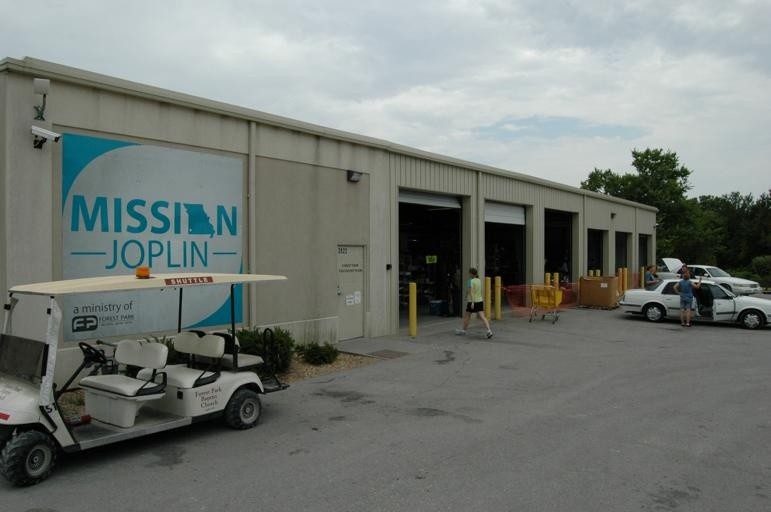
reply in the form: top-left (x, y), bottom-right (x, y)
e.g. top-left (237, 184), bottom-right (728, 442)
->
top-left (578, 273), bottom-right (619, 308)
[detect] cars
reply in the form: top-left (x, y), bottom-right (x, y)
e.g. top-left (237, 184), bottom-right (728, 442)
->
top-left (618, 278), bottom-right (770, 330)
top-left (655, 257), bottom-right (762, 295)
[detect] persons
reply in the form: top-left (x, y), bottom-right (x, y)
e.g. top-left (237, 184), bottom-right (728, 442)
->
top-left (644, 266), bottom-right (660, 290)
top-left (455, 267), bottom-right (493, 340)
top-left (682, 264), bottom-right (697, 279)
top-left (674, 272), bottom-right (702, 326)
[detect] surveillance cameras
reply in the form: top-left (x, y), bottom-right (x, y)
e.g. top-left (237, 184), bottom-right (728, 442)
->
top-left (656, 223), bottom-right (660, 227)
top-left (31, 126), bottom-right (62, 143)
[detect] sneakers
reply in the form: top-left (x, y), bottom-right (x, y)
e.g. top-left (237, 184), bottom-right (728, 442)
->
top-left (487, 331), bottom-right (493, 338)
top-left (457, 329), bottom-right (466, 334)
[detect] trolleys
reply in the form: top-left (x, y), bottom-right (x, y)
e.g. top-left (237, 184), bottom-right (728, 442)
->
top-left (526, 284), bottom-right (563, 324)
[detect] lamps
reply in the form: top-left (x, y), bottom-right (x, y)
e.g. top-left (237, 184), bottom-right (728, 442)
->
top-left (29, 75), bottom-right (51, 121)
top-left (346, 169), bottom-right (363, 184)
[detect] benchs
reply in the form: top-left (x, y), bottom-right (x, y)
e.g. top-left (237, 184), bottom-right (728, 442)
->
top-left (81, 336), bottom-right (170, 395)
top-left (137, 329), bottom-right (225, 393)
top-left (184, 330), bottom-right (264, 370)
